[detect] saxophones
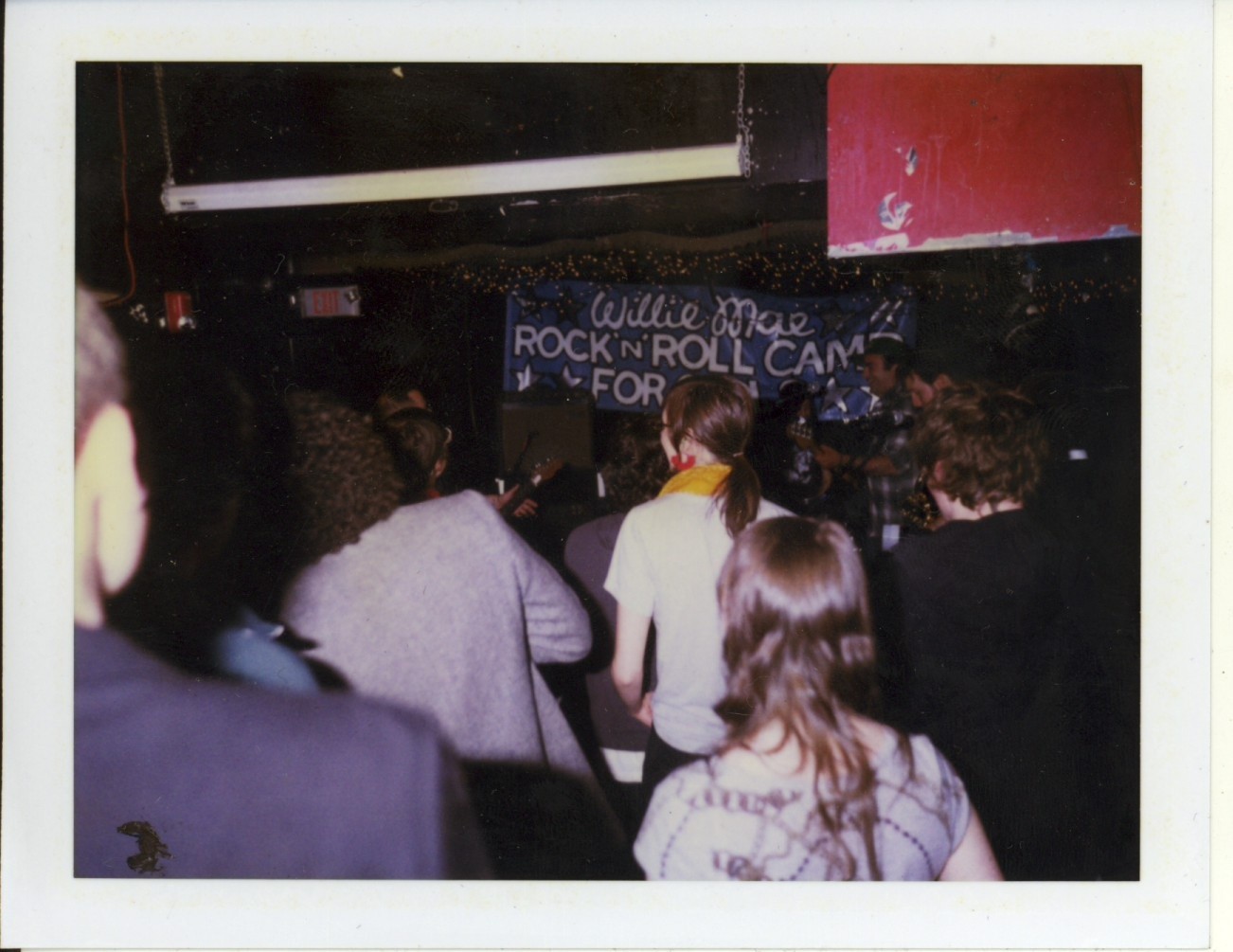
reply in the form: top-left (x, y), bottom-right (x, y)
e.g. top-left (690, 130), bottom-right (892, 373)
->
top-left (886, 462), bottom-right (943, 532)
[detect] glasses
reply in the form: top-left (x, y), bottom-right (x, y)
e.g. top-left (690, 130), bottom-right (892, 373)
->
top-left (651, 419), bottom-right (694, 439)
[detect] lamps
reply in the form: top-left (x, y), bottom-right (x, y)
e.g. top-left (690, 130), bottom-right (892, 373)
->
top-left (154, 61), bottom-right (751, 212)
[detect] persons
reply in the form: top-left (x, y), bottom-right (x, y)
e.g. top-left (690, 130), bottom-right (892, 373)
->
top-left (75, 278), bottom-right (1141, 881)
top-left (633, 518), bottom-right (1004, 881)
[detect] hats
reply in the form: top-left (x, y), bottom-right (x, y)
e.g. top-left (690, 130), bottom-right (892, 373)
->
top-left (846, 336), bottom-right (903, 368)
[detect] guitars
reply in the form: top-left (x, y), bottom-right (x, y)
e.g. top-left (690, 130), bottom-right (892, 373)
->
top-left (787, 404), bottom-right (863, 502)
top-left (498, 454), bottom-right (566, 519)
